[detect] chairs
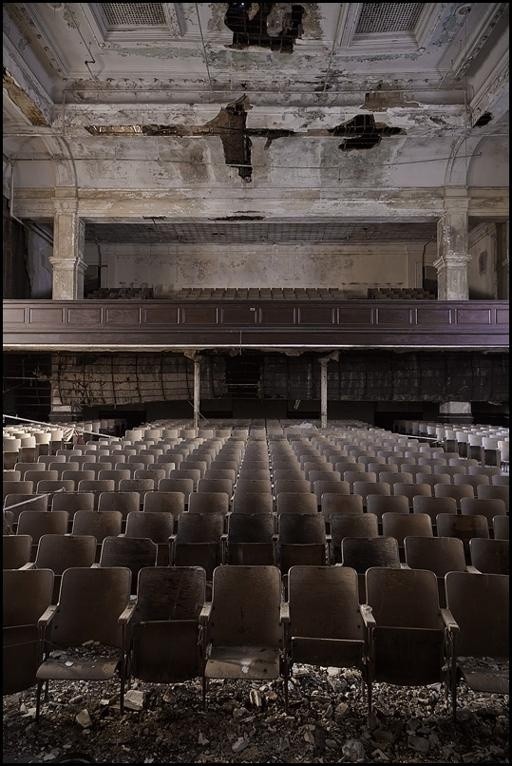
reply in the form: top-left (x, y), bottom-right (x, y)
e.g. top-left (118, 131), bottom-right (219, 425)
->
top-left (2, 417), bottom-right (154, 470)
top-left (122, 417), bottom-right (384, 435)
top-left (398, 415), bottom-right (510, 473)
top-left (173, 287), bottom-right (347, 301)
top-left (367, 287), bottom-right (435, 300)
